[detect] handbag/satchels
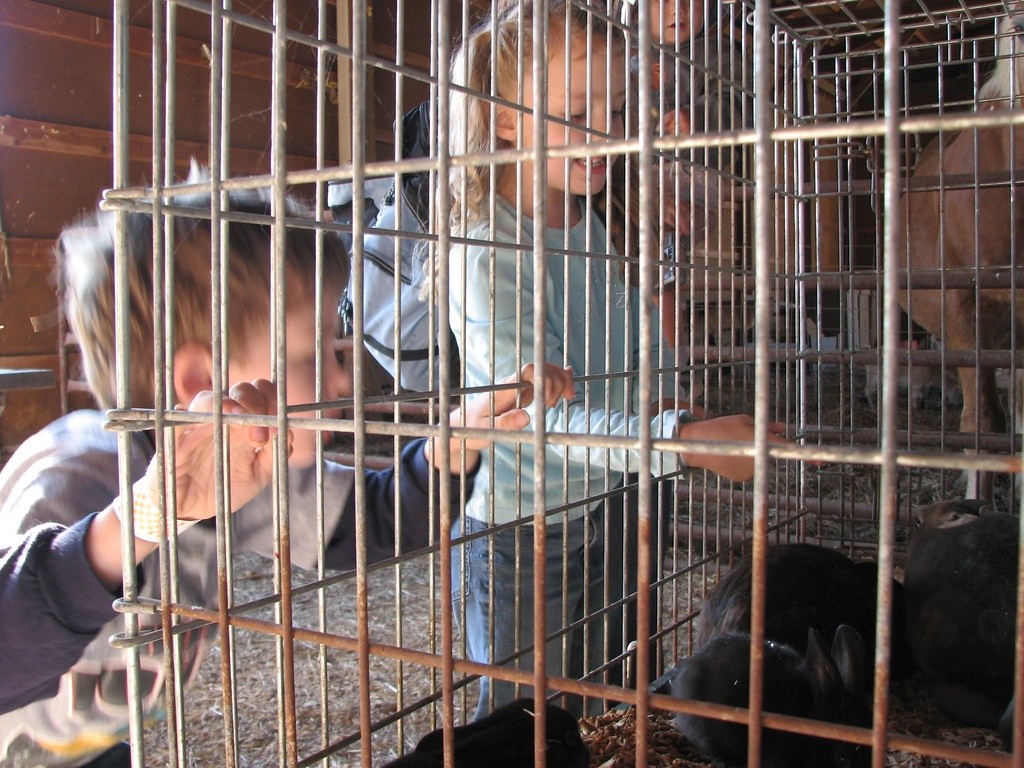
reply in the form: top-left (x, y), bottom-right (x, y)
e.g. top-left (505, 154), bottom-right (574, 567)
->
top-left (326, 178), bottom-right (462, 399)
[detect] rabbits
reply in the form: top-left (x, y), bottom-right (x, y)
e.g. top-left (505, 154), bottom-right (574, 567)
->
top-left (380, 498), bottom-right (1024, 768)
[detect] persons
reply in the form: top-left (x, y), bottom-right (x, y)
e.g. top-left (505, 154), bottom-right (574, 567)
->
top-left (431, 1), bottom-right (822, 723)
top-left (0, 157), bottom-right (578, 768)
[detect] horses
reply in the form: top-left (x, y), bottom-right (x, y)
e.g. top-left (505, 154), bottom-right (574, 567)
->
top-left (896, 1), bottom-right (1023, 507)
